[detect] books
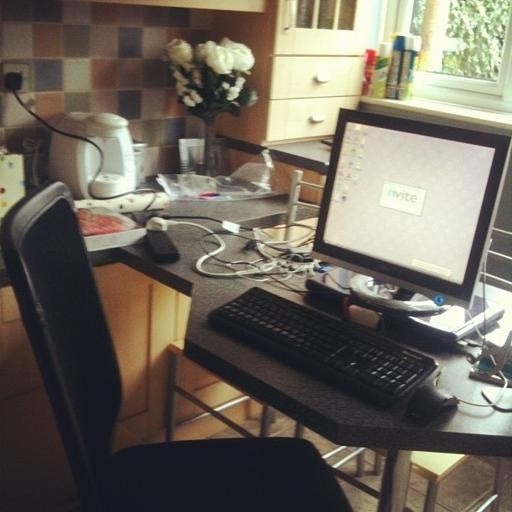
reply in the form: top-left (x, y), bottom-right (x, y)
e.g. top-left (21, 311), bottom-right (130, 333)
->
top-left (71, 205), bottom-right (150, 254)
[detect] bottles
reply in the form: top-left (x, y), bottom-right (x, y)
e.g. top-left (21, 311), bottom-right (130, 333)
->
top-left (360, 34), bottom-right (420, 102)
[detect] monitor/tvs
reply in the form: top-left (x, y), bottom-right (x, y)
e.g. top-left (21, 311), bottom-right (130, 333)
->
top-left (306, 106), bottom-right (512, 314)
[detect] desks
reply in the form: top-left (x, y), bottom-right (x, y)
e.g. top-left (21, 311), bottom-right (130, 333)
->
top-left (120, 195), bottom-right (512, 511)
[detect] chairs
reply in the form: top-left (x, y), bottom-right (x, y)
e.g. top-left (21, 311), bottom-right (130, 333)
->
top-left (0, 176), bottom-right (355, 511)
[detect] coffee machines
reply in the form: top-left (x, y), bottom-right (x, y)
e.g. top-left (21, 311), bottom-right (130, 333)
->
top-left (49, 110), bottom-right (139, 202)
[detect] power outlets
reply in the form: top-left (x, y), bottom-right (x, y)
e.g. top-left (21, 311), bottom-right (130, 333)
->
top-left (0, 62), bottom-right (30, 93)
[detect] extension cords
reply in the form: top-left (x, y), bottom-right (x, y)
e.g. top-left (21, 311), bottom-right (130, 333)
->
top-left (132, 209), bottom-right (179, 264)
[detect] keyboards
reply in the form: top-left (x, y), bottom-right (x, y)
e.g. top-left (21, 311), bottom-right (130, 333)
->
top-left (209, 286), bottom-right (440, 410)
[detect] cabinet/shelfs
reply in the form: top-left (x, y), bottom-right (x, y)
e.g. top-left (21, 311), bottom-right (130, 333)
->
top-left (212, 0), bottom-right (371, 148)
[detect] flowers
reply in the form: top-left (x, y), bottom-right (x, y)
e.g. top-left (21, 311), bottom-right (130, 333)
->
top-left (163, 35), bottom-right (259, 121)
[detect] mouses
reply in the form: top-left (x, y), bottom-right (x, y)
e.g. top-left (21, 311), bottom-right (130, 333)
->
top-left (406, 384), bottom-right (460, 427)
